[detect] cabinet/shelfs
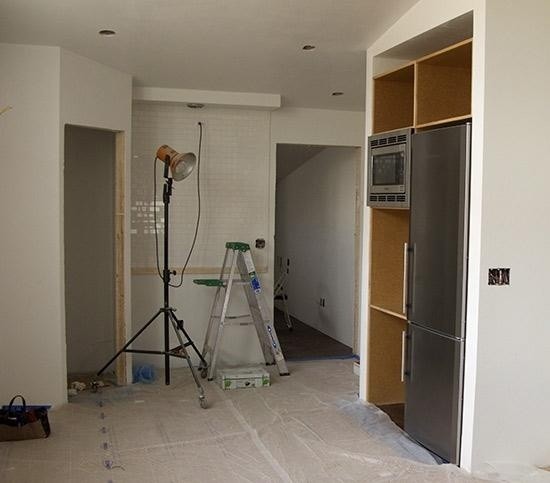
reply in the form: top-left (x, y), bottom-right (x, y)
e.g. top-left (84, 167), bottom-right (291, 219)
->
top-left (366, 38), bottom-right (474, 406)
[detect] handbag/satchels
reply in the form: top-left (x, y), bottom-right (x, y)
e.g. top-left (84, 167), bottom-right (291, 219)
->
top-left (0, 406), bottom-right (50, 440)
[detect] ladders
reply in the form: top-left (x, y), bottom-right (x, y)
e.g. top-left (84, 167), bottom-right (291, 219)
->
top-left (198, 242), bottom-right (290, 382)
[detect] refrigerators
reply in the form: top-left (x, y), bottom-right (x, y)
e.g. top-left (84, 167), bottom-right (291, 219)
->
top-left (399, 121), bottom-right (472, 468)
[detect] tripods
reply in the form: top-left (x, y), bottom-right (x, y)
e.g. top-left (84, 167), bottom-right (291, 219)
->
top-left (90, 185), bottom-right (209, 409)
